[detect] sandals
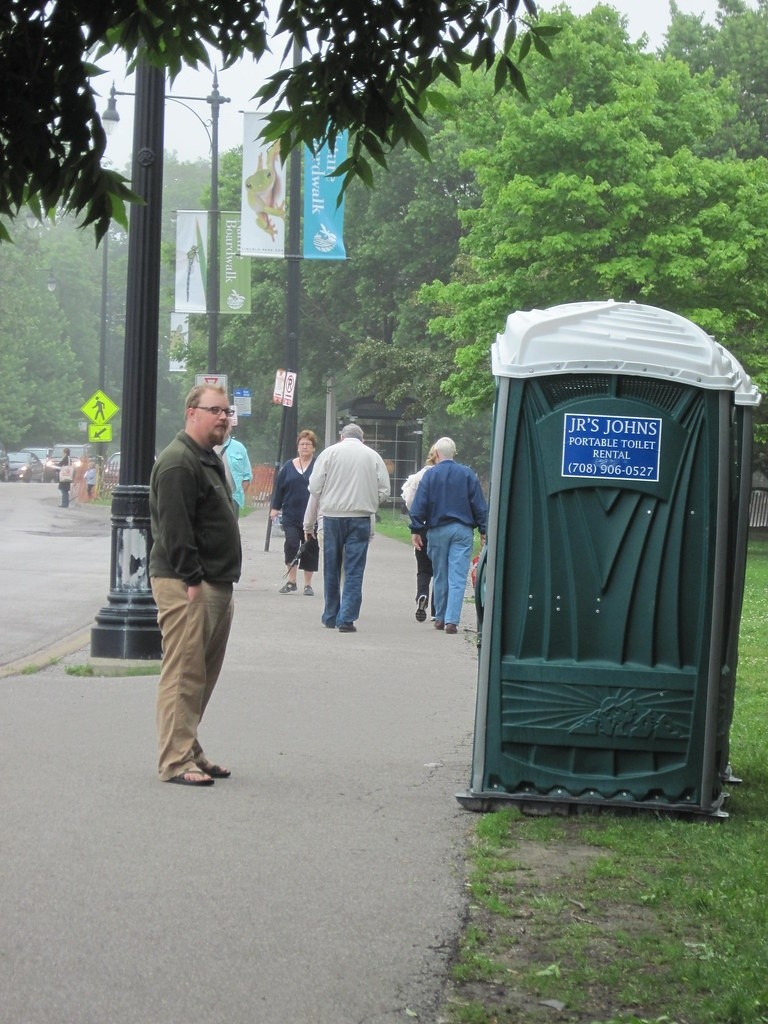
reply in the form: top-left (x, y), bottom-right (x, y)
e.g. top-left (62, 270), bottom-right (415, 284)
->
top-left (279, 581), bottom-right (297, 593)
top-left (303, 586), bottom-right (314, 595)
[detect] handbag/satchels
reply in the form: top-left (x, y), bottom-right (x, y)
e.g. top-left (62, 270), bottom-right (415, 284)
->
top-left (297, 534), bottom-right (319, 571)
top-left (59, 465), bottom-right (74, 483)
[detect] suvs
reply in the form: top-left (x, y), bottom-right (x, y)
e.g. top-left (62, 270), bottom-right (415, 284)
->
top-left (46, 441), bottom-right (95, 482)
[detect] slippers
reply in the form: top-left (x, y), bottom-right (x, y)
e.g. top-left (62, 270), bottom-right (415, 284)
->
top-left (166, 768), bottom-right (214, 784)
top-left (202, 764), bottom-right (231, 778)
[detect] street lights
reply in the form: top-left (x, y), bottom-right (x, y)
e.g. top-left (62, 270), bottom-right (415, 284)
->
top-left (101, 78), bottom-right (222, 373)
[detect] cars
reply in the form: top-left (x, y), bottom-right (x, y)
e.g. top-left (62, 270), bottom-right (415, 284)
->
top-left (20, 448), bottom-right (52, 482)
top-left (105, 451), bottom-right (122, 482)
top-left (7, 452), bottom-right (43, 482)
top-left (0, 442), bottom-right (10, 482)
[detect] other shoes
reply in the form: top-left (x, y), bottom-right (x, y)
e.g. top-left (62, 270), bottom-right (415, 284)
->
top-left (339, 625), bottom-right (357, 632)
top-left (430, 616), bottom-right (435, 621)
top-left (434, 621), bottom-right (445, 630)
top-left (415, 594), bottom-right (428, 622)
top-left (58, 503), bottom-right (68, 507)
top-left (326, 625), bottom-right (335, 628)
top-left (445, 623), bottom-right (457, 633)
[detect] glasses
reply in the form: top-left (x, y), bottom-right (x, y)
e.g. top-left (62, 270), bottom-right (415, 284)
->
top-left (188, 405), bottom-right (235, 417)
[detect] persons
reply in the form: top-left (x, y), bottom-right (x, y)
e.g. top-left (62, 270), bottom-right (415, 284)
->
top-left (84, 462), bottom-right (95, 499)
top-left (147, 387), bottom-right (243, 788)
top-left (305, 424), bottom-right (391, 632)
top-left (270, 430), bottom-right (319, 595)
top-left (55, 449), bottom-right (72, 508)
top-left (215, 425), bottom-right (253, 523)
top-left (403, 437), bottom-right (488, 633)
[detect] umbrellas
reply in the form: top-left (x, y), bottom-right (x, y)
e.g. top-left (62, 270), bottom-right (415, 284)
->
top-left (289, 533), bottom-right (316, 568)
